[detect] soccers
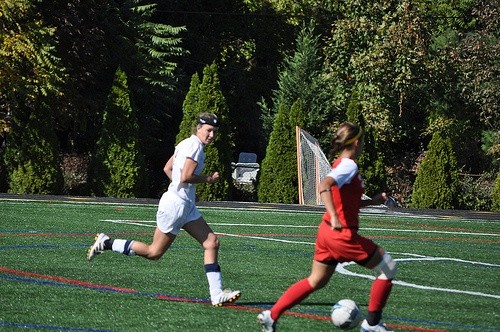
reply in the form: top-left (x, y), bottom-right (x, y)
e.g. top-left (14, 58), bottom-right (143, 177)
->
top-left (329, 298), bottom-right (363, 330)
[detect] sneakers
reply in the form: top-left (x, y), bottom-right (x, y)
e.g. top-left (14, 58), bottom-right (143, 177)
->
top-left (86, 232), bottom-right (108, 261)
top-left (360, 319), bottom-right (395, 332)
top-left (256, 309), bottom-right (274, 332)
top-left (211, 288), bottom-right (242, 307)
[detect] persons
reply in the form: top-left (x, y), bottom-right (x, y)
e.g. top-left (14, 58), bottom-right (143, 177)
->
top-left (258, 121), bottom-right (397, 332)
top-left (86, 112), bottom-right (241, 307)
